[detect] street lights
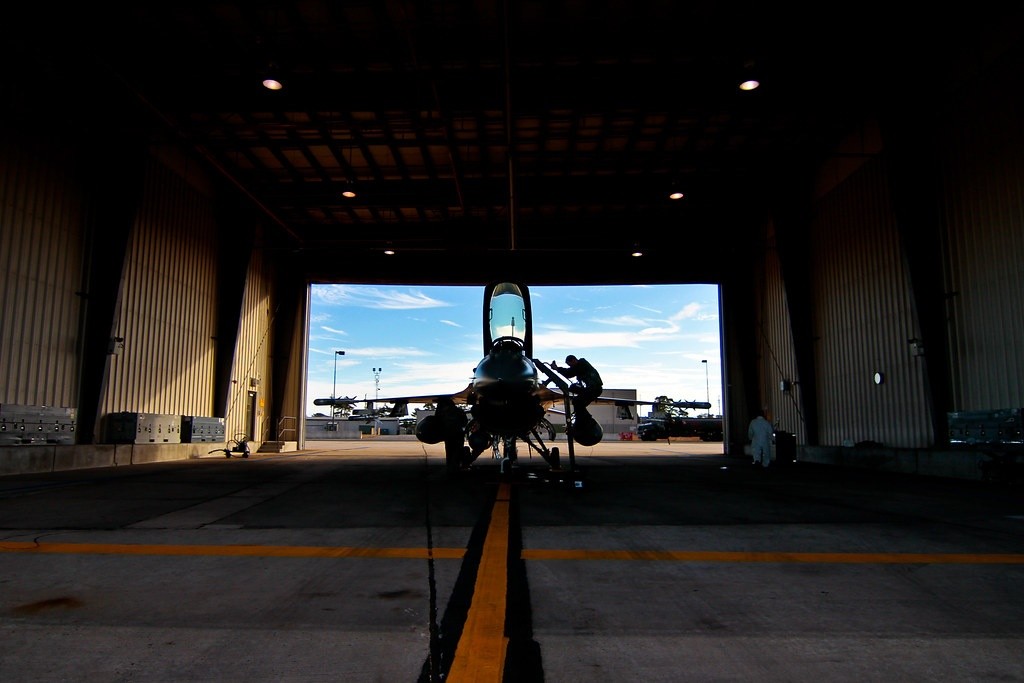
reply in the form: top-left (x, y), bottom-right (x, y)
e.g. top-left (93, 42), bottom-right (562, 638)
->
top-left (700, 359), bottom-right (711, 418)
top-left (372, 367), bottom-right (382, 409)
top-left (332, 351), bottom-right (345, 425)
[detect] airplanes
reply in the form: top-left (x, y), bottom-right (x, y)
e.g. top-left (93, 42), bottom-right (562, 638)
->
top-left (312, 276), bottom-right (711, 502)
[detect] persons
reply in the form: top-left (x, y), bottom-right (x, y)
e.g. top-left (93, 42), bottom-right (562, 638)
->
top-left (550, 355), bottom-right (603, 418)
top-left (748, 409), bottom-right (773, 467)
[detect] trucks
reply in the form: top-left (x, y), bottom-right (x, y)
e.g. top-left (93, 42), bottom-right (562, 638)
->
top-left (637, 411), bottom-right (723, 443)
